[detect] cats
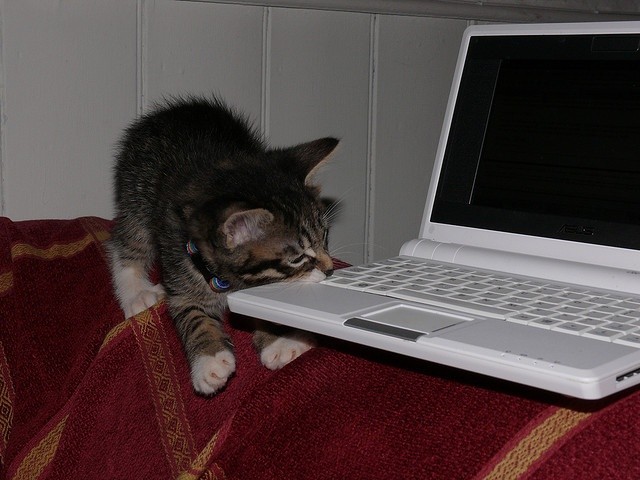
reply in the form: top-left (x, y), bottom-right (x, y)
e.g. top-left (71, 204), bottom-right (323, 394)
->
top-left (106, 95), bottom-right (343, 395)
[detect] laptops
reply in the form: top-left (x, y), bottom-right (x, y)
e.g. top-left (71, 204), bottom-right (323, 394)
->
top-left (226, 21), bottom-right (640, 404)
